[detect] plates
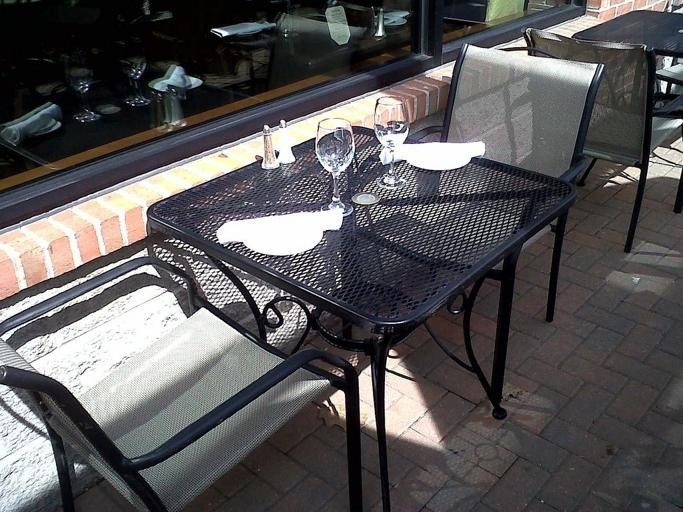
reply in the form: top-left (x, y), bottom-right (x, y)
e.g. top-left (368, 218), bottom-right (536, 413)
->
top-left (409, 140), bottom-right (471, 170)
top-left (243, 218), bottom-right (322, 256)
top-left (32, 115), bottom-right (62, 137)
top-left (147, 72), bottom-right (204, 91)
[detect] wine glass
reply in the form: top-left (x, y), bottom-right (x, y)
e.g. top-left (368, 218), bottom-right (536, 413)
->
top-left (312, 113), bottom-right (352, 219)
top-left (122, 41), bottom-right (148, 108)
top-left (66, 54), bottom-right (99, 122)
top-left (372, 96), bottom-right (408, 188)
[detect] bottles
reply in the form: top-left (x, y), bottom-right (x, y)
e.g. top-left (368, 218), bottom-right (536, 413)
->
top-left (365, 6), bottom-right (389, 41)
top-left (277, 28), bottom-right (300, 57)
top-left (149, 87), bottom-right (187, 133)
top-left (258, 119), bottom-right (296, 170)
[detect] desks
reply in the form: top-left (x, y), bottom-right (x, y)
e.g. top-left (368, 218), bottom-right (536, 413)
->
top-left (570, 6), bottom-right (682, 124)
top-left (137, 118), bottom-right (577, 511)
top-left (0, 43), bottom-right (257, 168)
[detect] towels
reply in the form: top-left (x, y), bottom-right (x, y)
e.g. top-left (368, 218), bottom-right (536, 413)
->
top-left (210, 207), bottom-right (346, 260)
top-left (373, 134), bottom-right (486, 174)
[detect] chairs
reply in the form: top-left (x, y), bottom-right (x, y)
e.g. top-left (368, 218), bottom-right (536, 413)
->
top-left (643, 0), bottom-right (683, 120)
top-left (518, 25), bottom-right (682, 253)
top-left (0, 256), bottom-right (364, 510)
top-left (260, 11), bottom-right (367, 97)
top-left (342, 36), bottom-right (610, 332)
